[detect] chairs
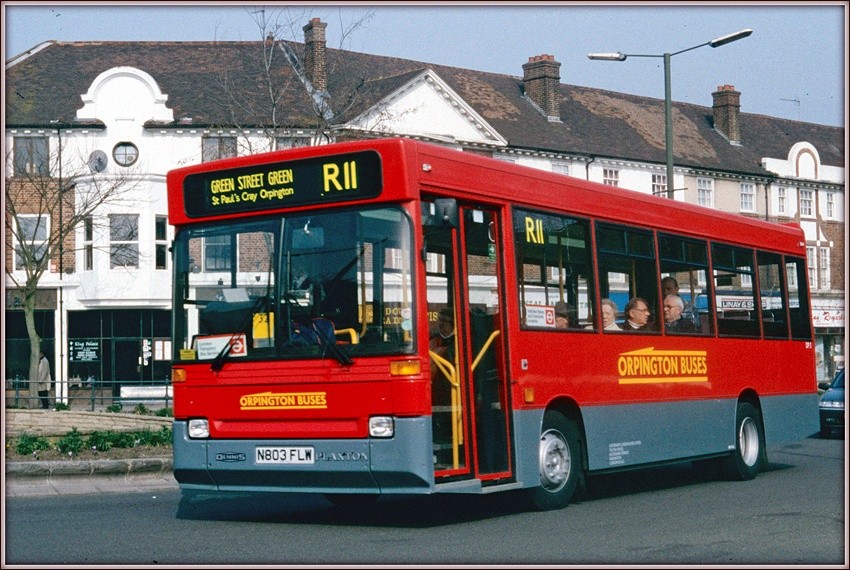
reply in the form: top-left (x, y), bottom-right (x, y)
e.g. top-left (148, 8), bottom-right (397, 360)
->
top-left (681, 305), bottom-right (775, 333)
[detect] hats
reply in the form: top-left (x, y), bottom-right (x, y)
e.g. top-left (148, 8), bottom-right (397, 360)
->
top-left (555, 302), bottom-right (574, 318)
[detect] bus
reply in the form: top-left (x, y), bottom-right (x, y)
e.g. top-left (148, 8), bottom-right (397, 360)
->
top-left (163, 137), bottom-right (819, 512)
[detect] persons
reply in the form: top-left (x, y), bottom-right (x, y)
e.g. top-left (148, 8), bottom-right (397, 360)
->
top-left (36, 351), bottom-right (52, 409)
top-left (428, 308), bottom-right (455, 402)
top-left (619, 296), bottom-right (654, 332)
top-left (584, 298), bottom-right (623, 331)
top-left (652, 294), bottom-right (699, 334)
top-left (660, 277), bottom-right (702, 329)
top-left (553, 301), bottom-right (583, 329)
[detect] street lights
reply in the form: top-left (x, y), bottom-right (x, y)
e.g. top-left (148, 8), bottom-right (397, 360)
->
top-left (587, 28), bottom-right (754, 202)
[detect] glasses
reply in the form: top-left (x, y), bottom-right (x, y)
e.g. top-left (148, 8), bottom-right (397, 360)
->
top-left (664, 304), bottom-right (677, 309)
top-left (633, 308), bottom-right (648, 312)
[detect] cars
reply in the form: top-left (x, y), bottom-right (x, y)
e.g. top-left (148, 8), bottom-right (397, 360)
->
top-left (817, 367), bottom-right (846, 439)
